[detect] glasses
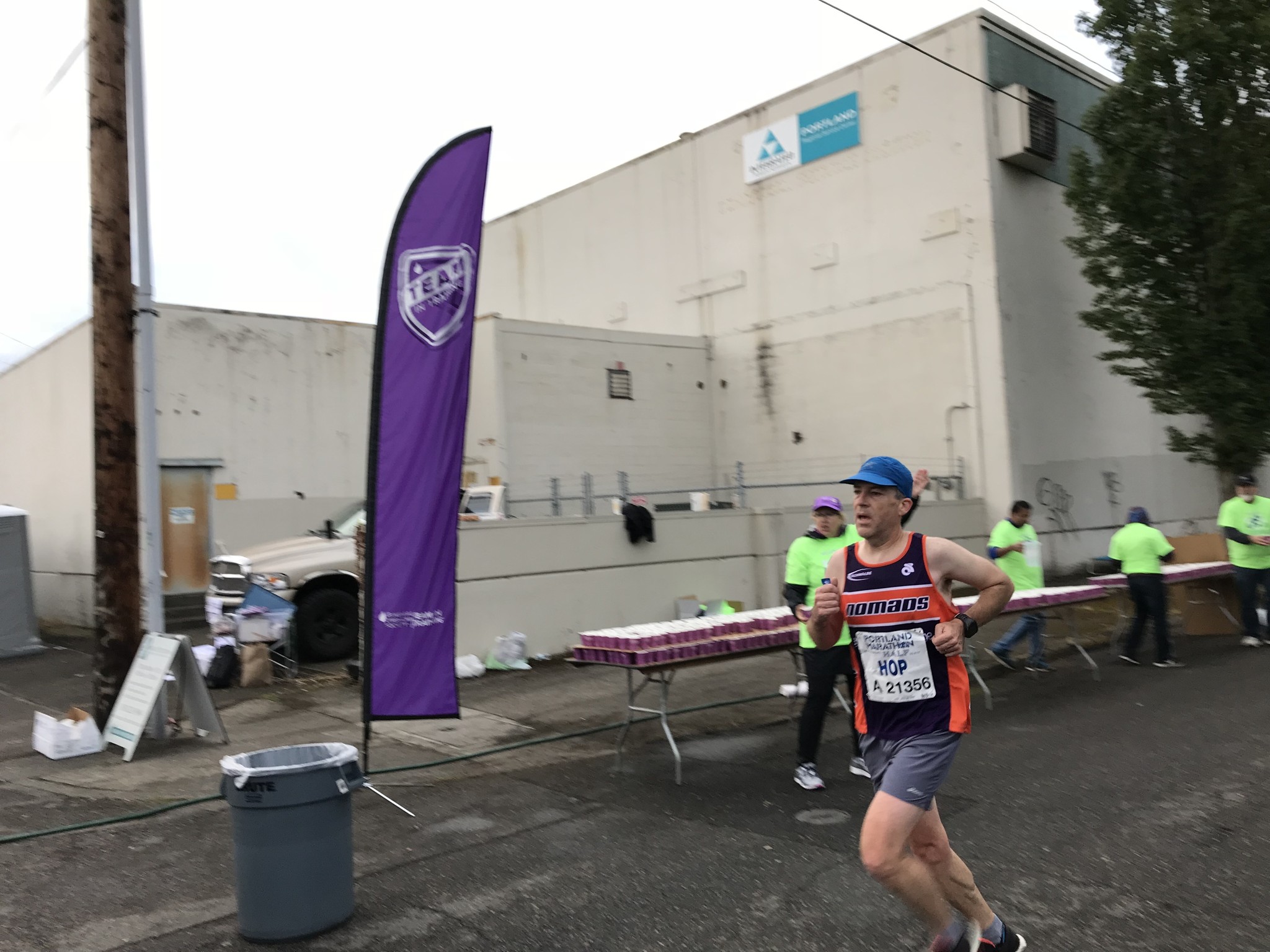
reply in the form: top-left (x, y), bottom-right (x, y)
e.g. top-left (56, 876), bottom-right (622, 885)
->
top-left (811, 511), bottom-right (838, 518)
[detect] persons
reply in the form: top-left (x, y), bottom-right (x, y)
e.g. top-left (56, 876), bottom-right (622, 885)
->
top-left (985, 500), bottom-right (1056, 673)
top-left (807, 456), bottom-right (1027, 952)
top-left (781, 470), bottom-right (930, 791)
top-left (1216, 474), bottom-right (1270, 647)
top-left (1108, 506), bottom-right (1185, 668)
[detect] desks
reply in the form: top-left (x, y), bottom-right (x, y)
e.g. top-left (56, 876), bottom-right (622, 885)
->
top-left (563, 557), bottom-right (1236, 785)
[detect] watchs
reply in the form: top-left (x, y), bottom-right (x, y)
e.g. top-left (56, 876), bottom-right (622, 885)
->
top-left (953, 612), bottom-right (979, 639)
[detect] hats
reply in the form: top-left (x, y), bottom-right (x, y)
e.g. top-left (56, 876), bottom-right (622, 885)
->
top-left (1233, 474), bottom-right (1255, 486)
top-left (812, 495), bottom-right (843, 512)
top-left (838, 456), bottom-right (913, 498)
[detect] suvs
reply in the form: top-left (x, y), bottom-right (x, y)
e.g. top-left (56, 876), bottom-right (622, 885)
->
top-left (204, 486), bottom-right (508, 663)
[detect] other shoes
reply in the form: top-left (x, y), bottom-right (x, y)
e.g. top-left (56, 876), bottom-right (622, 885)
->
top-left (978, 923), bottom-right (1027, 952)
top-left (1264, 636), bottom-right (1270, 644)
top-left (1025, 661), bottom-right (1056, 672)
top-left (930, 918), bottom-right (981, 952)
top-left (984, 646), bottom-right (1016, 670)
top-left (1119, 653), bottom-right (1141, 665)
top-left (1241, 635), bottom-right (1264, 647)
top-left (1151, 656), bottom-right (1186, 668)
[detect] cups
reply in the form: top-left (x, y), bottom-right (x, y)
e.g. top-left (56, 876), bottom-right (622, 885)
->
top-left (800, 606), bottom-right (813, 618)
top-left (1087, 561), bottom-right (1234, 584)
top-left (572, 605), bottom-right (799, 665)
top-left (952, 584), bottom-right (1104, 614)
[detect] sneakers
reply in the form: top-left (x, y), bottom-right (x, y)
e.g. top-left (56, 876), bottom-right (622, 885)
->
top-left (793, 761), bottom-right (825, 790)
top-left (849, 755), bottom-right (871, 779)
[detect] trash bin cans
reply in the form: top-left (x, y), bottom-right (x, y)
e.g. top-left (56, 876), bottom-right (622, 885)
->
top-left (218, 744), bottom-right (366, 943)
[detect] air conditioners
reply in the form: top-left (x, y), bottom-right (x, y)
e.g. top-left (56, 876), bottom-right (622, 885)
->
top-left (997, 82), bottom-right (1059, 168)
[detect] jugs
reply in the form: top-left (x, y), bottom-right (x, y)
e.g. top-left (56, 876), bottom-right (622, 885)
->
top-left (1019, 540), bottom-right (1042, 567)
